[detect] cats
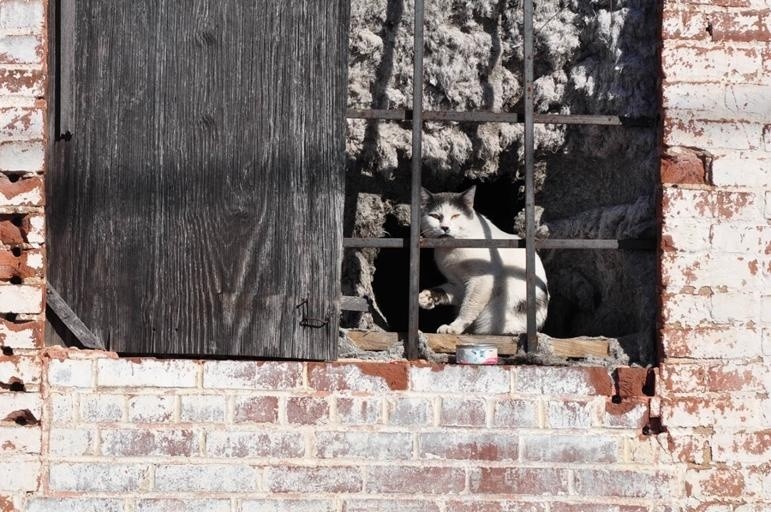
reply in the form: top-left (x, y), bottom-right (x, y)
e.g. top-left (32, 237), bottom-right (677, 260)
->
top-left (418, 185), bottom-right (552, 336)
